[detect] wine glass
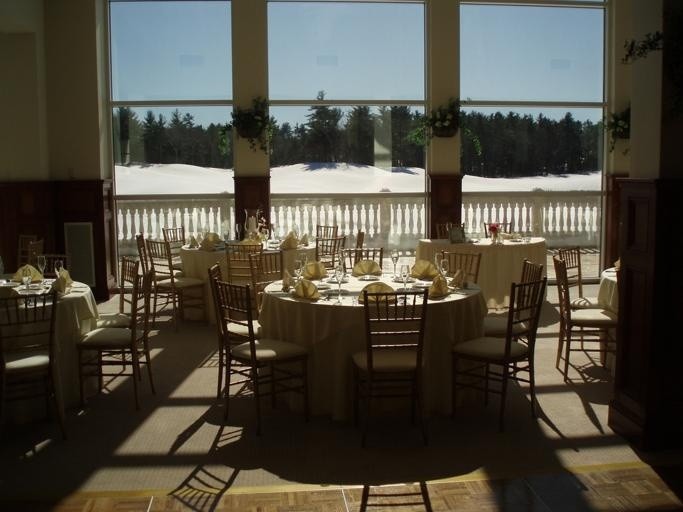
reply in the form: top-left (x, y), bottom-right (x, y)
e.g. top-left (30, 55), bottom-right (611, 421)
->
top-left (22, 270), bottom-right (33, 305)
top-left (338, 246), bottom-right (345, 269)
top-left (390, 251), bottom-right (398, 277)
top-left (335, 266), bottom-right (343, 302)
top-left (442, 260), bottom-right (448, 273)
top-left (37, 255), bottom-right (45, 277)
top-left (224, 223), bottom-right (269, 243)
top-left (293, 254), bottom-right (305, 277)
top-left (400, 264), bottom-right (410, 290)
top-left (54, 260), bottom-right (64, 278)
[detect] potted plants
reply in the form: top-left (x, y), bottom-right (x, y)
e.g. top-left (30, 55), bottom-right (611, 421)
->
top-left (217, 94), bottom-right (277, 156)
top-left (401, 96), bottom-right (483, 157)
